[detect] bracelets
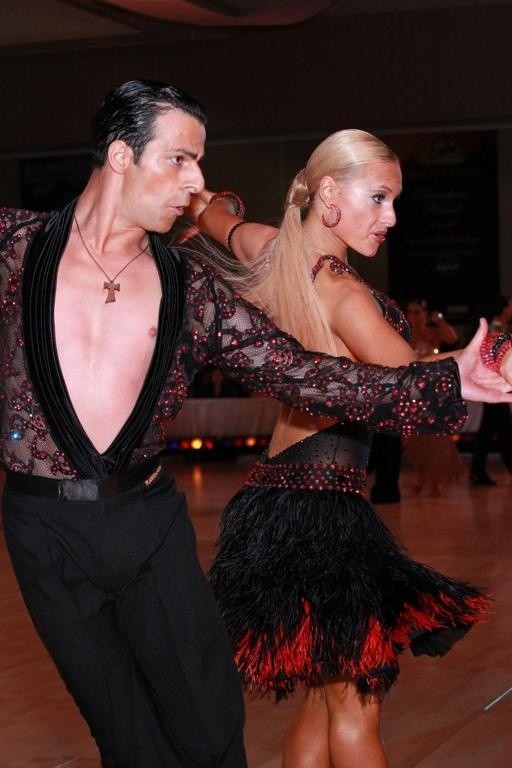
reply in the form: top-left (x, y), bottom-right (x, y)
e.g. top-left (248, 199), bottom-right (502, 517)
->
top-left (207, 190), bottom-right (246, 218)
top-left (479, 330), bottom-right (512, 373)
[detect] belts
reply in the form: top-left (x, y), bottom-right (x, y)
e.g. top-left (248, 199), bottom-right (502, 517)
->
top-left (6, 450), bottom-right (160, 502)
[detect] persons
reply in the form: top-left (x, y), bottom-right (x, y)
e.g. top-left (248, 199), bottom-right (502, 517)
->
top-left (397, 297), bottom-right (470, 501)
top-left (0, 78), bottom-right (512, 768)
top-left (468, 295), bottom-right (512, 487)
top-left (168, 127), bottom-right (512, 768)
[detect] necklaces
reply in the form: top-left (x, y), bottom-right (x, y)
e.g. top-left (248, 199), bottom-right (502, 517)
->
top-left (73, 213), bottom-right (150, 305)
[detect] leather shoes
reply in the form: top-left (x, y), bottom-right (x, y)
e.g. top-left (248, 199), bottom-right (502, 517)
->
top-left (468, 473), bottom-right (496, 486)
